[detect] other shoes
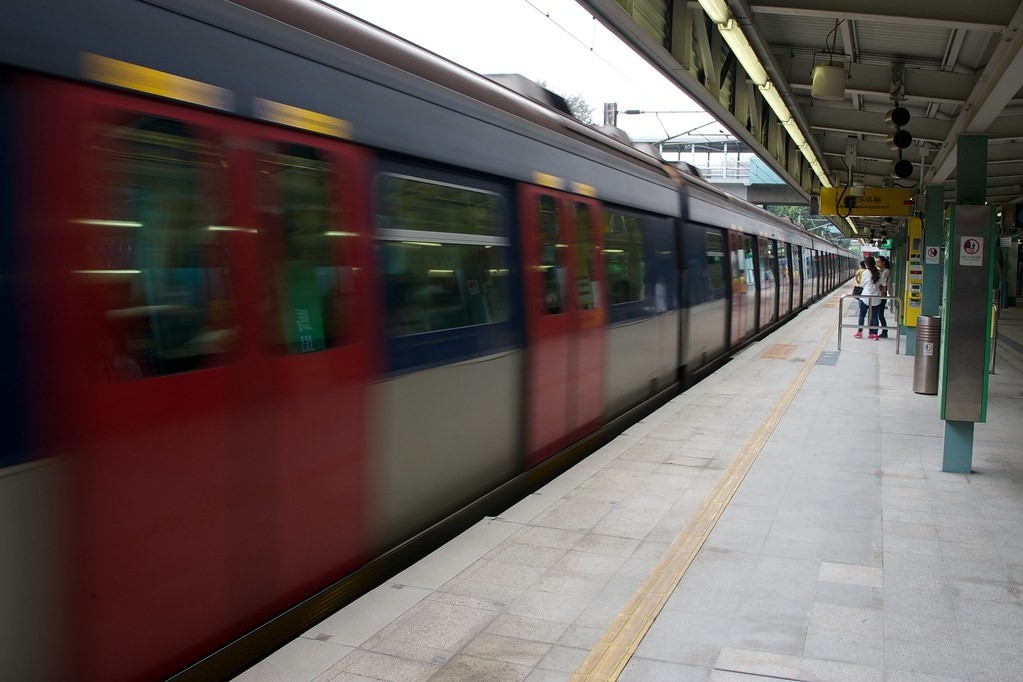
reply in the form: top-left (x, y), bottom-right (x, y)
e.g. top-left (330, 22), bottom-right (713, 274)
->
top-left (868, 333), bottom-right (878, 340)
top-left (878, 332), bottom-right (887, 338)
top-left (854, 331), bottom-right (862, 338)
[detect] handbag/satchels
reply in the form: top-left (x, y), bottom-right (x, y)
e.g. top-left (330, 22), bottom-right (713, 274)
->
top-left (852, 284), bottom-right (863, 300)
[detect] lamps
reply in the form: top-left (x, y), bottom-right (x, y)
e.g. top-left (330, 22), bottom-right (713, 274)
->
top-left (700, 0), bottom-right (858, 237)
top-left (889, 159), bottom-right (913, 179)
top-left (850, 174), bottom-right (867, 197)
top-left (887, 129), bottom-right (912, 150)
top-left (880, 218), bottom-right (892, 226)
top-left (885, 109), bottom-right (910, 127)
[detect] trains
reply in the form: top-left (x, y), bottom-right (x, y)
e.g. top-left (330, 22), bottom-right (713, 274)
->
top-left (0, 0), bottom-right (869, 682)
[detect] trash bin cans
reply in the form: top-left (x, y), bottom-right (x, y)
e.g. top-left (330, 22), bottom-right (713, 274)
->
top-left (912, 314), bottom-right (941, 395)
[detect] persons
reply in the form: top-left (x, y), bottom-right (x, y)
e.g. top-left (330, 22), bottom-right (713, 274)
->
top-left (733, 268), bottom-right (749, 307)
top-left (855, 256), bottom-right (891, 340)
